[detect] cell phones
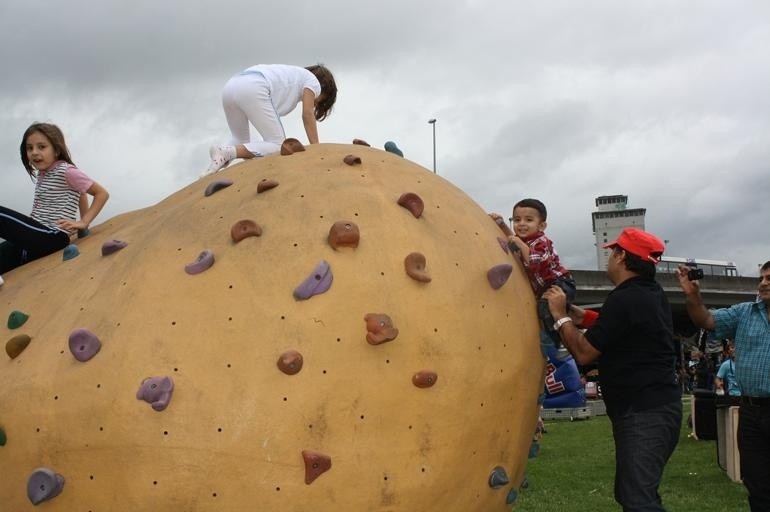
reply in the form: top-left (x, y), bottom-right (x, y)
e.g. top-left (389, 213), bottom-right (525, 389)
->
top-left (688, 268), bottom-right (704, 280)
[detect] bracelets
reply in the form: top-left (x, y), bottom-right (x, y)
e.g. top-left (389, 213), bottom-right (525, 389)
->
top-left (553, 317), bottom-right (572, 333)
top-left (582, 309), bottom-right (598, 328)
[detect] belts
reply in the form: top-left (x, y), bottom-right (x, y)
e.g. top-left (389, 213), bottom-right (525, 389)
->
top-left (738, 398), bottom-right (766, 409)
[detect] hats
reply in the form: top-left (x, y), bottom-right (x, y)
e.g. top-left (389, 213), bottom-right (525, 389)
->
top-left (603, 228), bottom-right (664, 265)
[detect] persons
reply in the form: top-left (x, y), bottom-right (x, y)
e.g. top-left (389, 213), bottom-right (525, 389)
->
top-left (490, 198), bottom-right (577, 351)
top-left (674, 259), bottom-right (769, 512)
top-left (541, 227), bottom-right (683, 512)
top-left (674, 307), bottom-right (741, 398)
top-left (0, 120), bottom-right (110, 286)
top-left (200, 62), bottom-right (338, 175)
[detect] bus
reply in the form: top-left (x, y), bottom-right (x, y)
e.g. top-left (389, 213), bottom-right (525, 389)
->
top-left (655, 257), bottom-right (737, 276)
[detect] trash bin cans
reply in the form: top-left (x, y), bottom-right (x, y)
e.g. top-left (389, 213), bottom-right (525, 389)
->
top-left (694, 390), bottom-right (743, 484)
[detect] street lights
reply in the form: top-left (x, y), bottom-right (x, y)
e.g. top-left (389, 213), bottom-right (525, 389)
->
top-left (428, 119), bottom-right (436, 173)
